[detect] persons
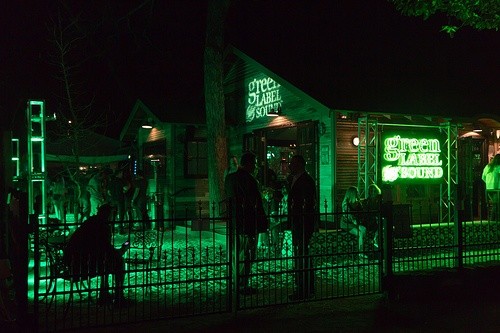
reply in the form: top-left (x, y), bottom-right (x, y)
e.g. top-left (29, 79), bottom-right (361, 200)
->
top-left (7, 160), bottom-right (153, 230)
top-left (223, 155), bottom-right (317, 301)
top-left (481, 153), bottom-right (500, 222)
top-left (62, 203), bottom-right (135, 309)
top-left (340, 184), bottom-right (384, 259)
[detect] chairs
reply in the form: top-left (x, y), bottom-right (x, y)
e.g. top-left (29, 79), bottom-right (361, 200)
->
top-left (32, 238), bottom-right (99, 304)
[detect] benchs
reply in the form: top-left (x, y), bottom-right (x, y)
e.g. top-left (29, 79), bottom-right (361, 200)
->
top-left (283, 220), bottom-right (500, 256)
